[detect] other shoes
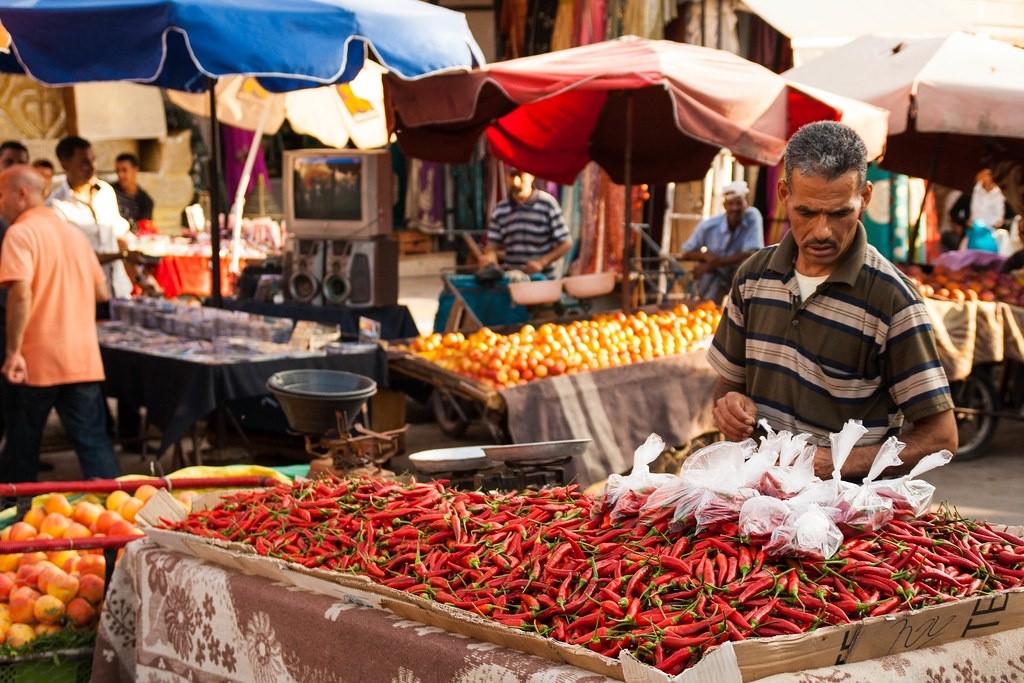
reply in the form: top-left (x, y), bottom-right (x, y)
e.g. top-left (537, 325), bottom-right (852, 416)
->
top-left (119, 441), bottom-right (157, 456)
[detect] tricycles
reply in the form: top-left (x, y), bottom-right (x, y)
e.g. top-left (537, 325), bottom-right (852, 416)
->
top-left (880, 265), bottom-right (1023, 458)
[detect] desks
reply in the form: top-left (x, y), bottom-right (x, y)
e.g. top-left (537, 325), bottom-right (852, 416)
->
top-left (89, 537), bottom-right (1023, 683)
top-left (93, 320), bottom-right (386, 469)
top-left (130, 237), bottom-right (271, 298)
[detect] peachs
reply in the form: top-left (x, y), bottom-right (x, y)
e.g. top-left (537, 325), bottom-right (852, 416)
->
top-left (0, 484), bottom-right (161, 649)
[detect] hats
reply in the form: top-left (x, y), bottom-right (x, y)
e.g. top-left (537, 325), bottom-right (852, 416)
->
top-left (505, 165), bottom-right (518, 174)
top-left (722, 182), bottom-right (749, 200)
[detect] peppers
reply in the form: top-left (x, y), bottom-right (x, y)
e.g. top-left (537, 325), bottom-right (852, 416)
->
top-left (154, 469), bottom-right (1023, 675)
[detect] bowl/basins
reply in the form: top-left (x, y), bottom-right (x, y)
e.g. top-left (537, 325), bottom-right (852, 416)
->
top-left (562, 271), bottom-right (619, 298)
top-left (265, 368), bottom-right (378, 434)
top-left (508, 280), bottom-right (563, 305)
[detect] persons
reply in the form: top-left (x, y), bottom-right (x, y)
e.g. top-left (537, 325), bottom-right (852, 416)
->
top-left (108, 152), bottom-right (154, 280)
top-left (0, 163), bottom-right (124, 529)
top-left (679, 181), bottom-right (765, 303)
top-left (0, 140), bottom-right (29, 246)
top-left (33, 158), bottom-right (68, 210)
top-left (45, 135), bottom-right (135, 320)
top-left (949, 168), bottom-right (1018, 234)
top-left (706, 120), bottom-right (959, 490)
top-left (483, 162), bottom-right (574, 282)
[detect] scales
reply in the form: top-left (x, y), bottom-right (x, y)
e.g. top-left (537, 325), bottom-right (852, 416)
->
top-left (266, 370), bottom-right (592, 491)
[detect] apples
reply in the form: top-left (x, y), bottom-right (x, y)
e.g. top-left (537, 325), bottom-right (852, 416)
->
top-left (894, 264), bottom-right (1024, 308)
top-left (410, 298), bottom-right (723, 390)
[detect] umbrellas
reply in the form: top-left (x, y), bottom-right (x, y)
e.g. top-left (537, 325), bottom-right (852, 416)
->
top-left (0, 0), bottom-right (487, 305)
top-left (167, 56), bottom-right (399, 272)
top-left (780, 28), bottom-right (1024, 241)
top-left (378, 37), bottom-right (890, 308)
